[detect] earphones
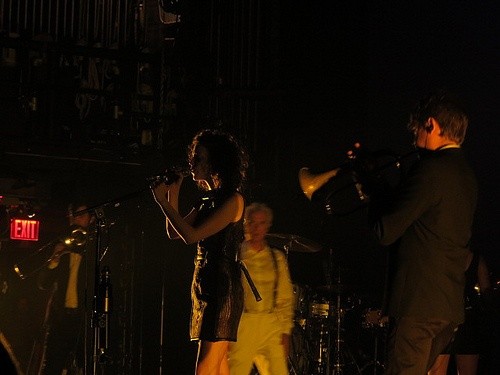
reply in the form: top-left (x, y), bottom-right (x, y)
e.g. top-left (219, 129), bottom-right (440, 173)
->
top-left (427, 122), bottom-right (433, 132)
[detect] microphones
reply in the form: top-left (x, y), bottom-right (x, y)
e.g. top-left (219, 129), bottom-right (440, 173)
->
top-left (144, 169), bottom-right (190, 184)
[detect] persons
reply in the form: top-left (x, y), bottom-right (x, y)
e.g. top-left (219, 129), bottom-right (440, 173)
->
top-left (0, 91), bottom-right (500, 375)
top-left (147, 128), bottom-right (263, 375)
top-left (228, 202), bottom-right (298, 375)
